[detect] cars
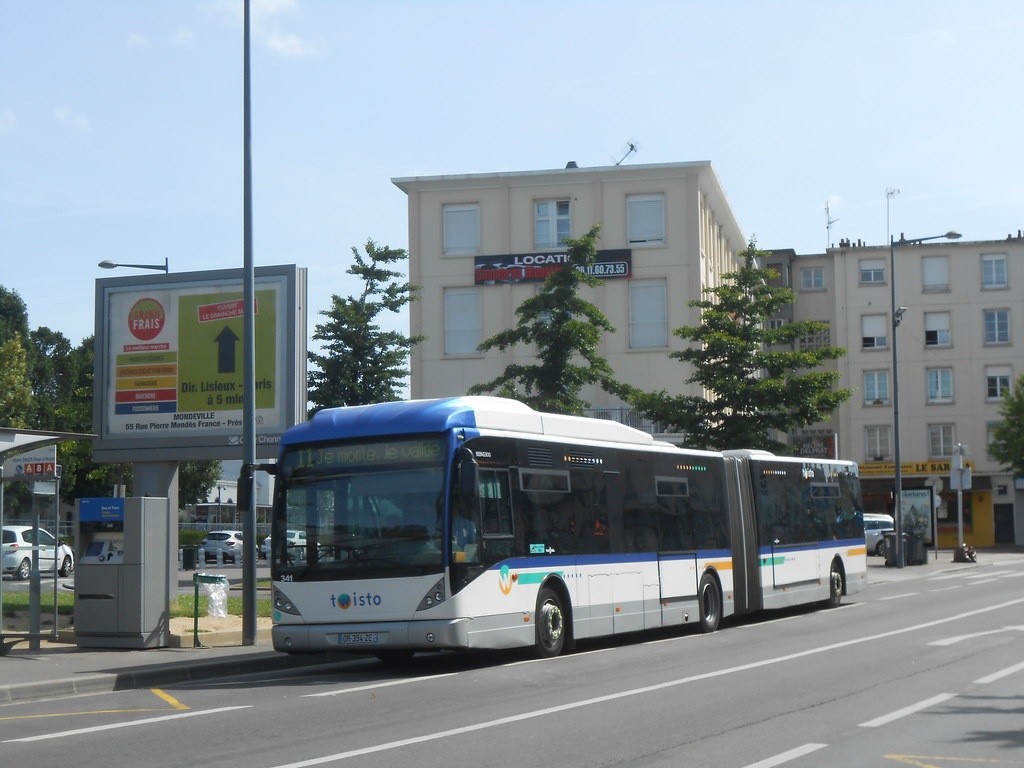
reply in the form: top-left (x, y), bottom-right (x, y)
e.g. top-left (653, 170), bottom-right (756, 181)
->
top-left (260, 530), bottom-right (323, 560)
top-left (201, 530), bottom-right (260, 562)
top-left (2, 525), bottom-right (73, 580)
top-left (860, 513), bottom-right (894, 556)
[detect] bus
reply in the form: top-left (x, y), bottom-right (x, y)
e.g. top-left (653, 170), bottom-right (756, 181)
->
top-left (237, 394), bottom-right (869, 658)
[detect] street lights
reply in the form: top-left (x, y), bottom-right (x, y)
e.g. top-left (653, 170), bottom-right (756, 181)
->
top-left (887, 230), bottom-right (965, 567)
top-left (98, 256), bottom-right (170, 273)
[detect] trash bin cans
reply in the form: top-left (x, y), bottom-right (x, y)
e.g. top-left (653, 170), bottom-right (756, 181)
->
top-left (904, 526), bottom-right (927, 566)
top-left (881, 530), bottom-right (908, 567)
